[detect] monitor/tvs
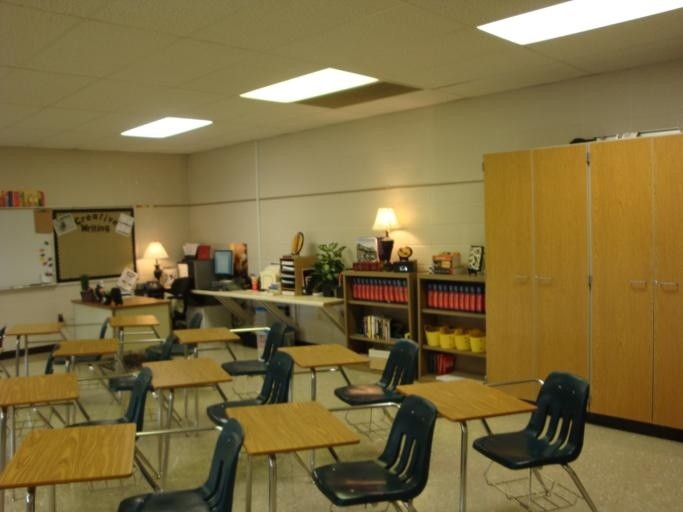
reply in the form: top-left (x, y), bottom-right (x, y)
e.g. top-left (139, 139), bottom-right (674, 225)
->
top-left (213, 250), bottom-right (234, 277)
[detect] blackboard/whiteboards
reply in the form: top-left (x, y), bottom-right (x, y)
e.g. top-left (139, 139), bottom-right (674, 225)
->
top-left (0, 207), bottom-right (136, 291)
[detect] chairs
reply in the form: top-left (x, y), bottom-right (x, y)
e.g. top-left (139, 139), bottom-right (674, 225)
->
top-left (0, 313), bottom-right (599, 512)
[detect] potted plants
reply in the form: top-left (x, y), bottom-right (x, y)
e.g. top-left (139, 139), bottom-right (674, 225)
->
top-left (313, 241), bottom-right (347, 297)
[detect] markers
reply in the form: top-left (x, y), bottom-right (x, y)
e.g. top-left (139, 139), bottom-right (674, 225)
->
top-left (30, 283), bottom-right (41, 286)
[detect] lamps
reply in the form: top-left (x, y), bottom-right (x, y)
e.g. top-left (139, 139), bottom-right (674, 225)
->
top-left (143, 241), bottom-right (169, 281)
top-left (371, 206), bottom-right (402, 262)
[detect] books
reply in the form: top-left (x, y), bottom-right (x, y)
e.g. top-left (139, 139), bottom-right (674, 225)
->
top-left (346, 276), bottom-right (408, 342)
top-left (423, 280), bottom-right (484, 312)
top-left (424, 349), bottom-right (454, 375)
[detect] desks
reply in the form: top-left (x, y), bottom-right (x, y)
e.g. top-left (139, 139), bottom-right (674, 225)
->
top-left (191, 287), bottom-right (345, 335)
top-left (71, 295), bottom-right (172, 360)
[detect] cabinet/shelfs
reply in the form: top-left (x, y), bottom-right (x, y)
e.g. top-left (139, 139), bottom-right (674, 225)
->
top-left (278, 253), bottom-right (319, 297)
top-left (482, 141), bottom-right (589, 423)
top-left (587, 133), bottom-right (682, 441)
top-left (415, 273), bottom-right (485, 385)
top-left (341, 270), bottom-right (421, 381)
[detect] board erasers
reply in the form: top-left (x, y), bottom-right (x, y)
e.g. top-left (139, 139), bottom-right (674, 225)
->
top-left (11, 285), bottom-right (23, 289)
